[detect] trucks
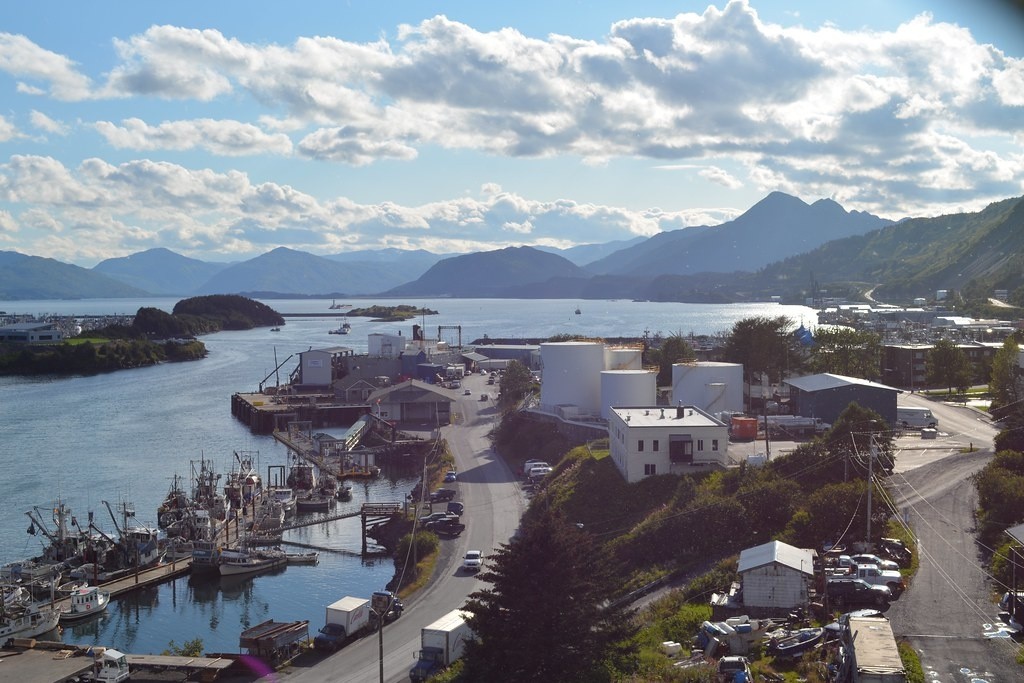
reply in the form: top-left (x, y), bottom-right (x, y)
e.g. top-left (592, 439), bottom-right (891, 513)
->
top-left (370, 589), bottom-right (404, 626)
top-left (312, 595), bottom-right (371, 652)
top-left (767, 418), bottom-right (832, 436)
top-left (417, 502), bottom-right (465, 535)
top-left (431, 488), bottom-right (457, 501)
top-left (407, 609), bottom-right (480, 676)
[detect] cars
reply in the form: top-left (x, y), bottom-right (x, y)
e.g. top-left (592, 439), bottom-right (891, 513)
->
top-left (998, 592), bottom-right (1024, 609)
top-left (446, 364), bottom-right (505, 401)
top-left (525, 459), bottom-right (552, 481)
top-left (445, 470), bottom-right (458, 480)
top-left (462, 550), bottom-right (489, 569)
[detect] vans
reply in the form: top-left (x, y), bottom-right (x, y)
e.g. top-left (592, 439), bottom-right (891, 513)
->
top-left (895, 407), bottom-right (939, 429)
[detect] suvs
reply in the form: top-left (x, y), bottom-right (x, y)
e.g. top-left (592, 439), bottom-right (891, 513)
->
top-left (825, 609), bottom-right (889, 640)
top-left (819, 552), bottom-right (905, 603)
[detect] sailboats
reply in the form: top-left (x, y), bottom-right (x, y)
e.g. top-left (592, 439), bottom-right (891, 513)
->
top-left (1008, 545), bottom-right (1024, 634)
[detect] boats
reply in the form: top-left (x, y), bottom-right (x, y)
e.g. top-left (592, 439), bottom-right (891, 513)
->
top-left (0, 447), bottom-right (357, 646)
top-left (693, 608), bottom-right (825, 683)
top-left (329, 316), bottom-right (353, 336)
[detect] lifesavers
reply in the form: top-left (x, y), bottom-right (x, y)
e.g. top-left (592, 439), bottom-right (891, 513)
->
top-left (324, 449), bottom-right (329, 456)
top-left (86, 604), bottom-right (90, 609)
